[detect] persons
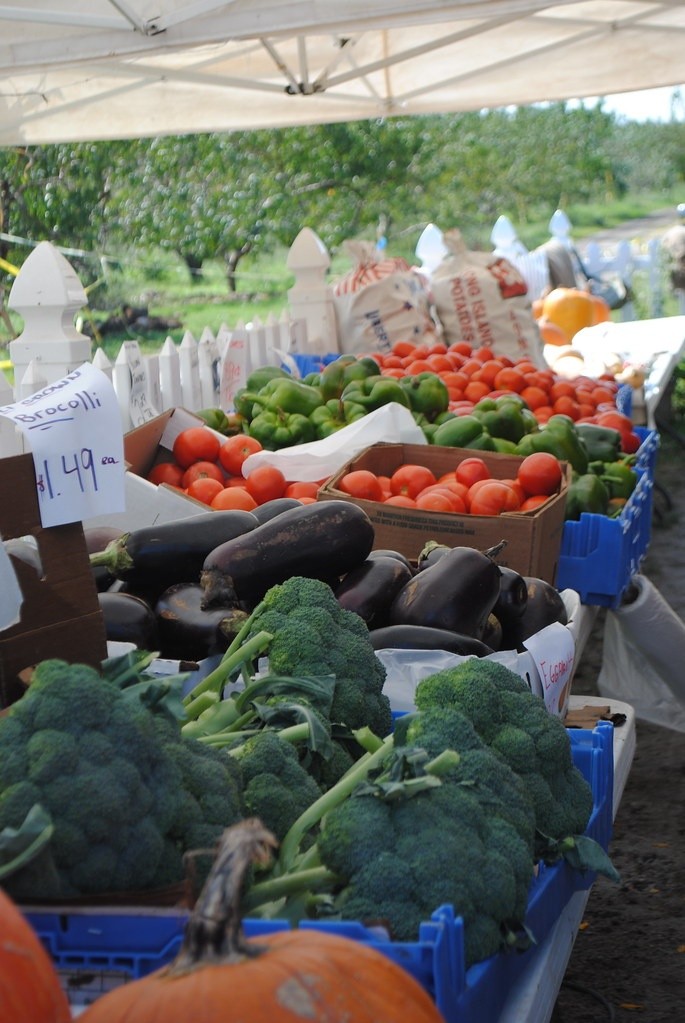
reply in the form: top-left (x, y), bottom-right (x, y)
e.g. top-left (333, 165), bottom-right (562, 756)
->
top-left (122, 303), bottom-right (179, 331)
top-left (660, 203), bottom-right (685, 290)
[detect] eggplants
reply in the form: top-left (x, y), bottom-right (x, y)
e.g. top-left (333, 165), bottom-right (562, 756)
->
top-left (84, 498), bottom-right (568, 661)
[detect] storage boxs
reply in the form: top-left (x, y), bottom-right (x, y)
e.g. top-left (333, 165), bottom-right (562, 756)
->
top-left (1, 381), bottom-right (661, 1020)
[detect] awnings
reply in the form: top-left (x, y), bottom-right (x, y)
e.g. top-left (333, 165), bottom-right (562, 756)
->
top-left (0, 0), bottom-right (684, 154)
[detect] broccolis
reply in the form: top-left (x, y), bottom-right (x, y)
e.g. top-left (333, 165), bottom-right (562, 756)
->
top-left (0, 574), bottom-right (620, 968)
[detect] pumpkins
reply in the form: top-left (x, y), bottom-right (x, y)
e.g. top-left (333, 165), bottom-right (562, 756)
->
top-left (531, 287), bottom-right (613, 344)
top-left (0, 817), bottom-right (445, 1023)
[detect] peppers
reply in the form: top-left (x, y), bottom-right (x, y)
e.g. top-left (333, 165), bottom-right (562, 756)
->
top-left (194, 354), bottom-right (640, 522)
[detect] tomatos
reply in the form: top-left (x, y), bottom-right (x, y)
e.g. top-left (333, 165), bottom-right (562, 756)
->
top-left (144, 425), bottom-right (562, 518)
top-left (362, 342), bottom-right (643, 451)
